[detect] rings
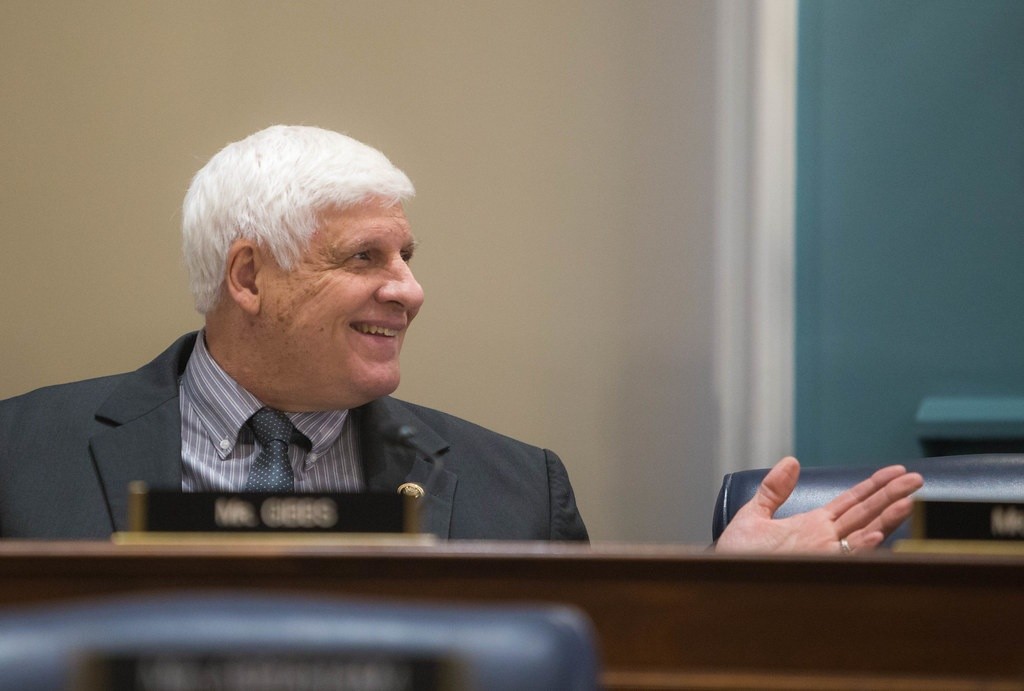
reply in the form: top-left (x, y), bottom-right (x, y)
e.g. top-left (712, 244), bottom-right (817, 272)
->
top-left (841, 539), bottom-right (851, 552)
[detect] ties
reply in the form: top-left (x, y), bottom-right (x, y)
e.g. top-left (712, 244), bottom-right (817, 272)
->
top-left (243, 409), bottom-right (296, 493)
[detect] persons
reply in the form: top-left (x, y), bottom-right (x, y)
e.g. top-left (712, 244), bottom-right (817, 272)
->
top-left (1, 124), bottom-right (925, 554)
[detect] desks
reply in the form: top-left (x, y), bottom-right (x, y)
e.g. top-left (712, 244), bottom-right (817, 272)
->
top-left (0, 537), bottom-right (1024, 691)
